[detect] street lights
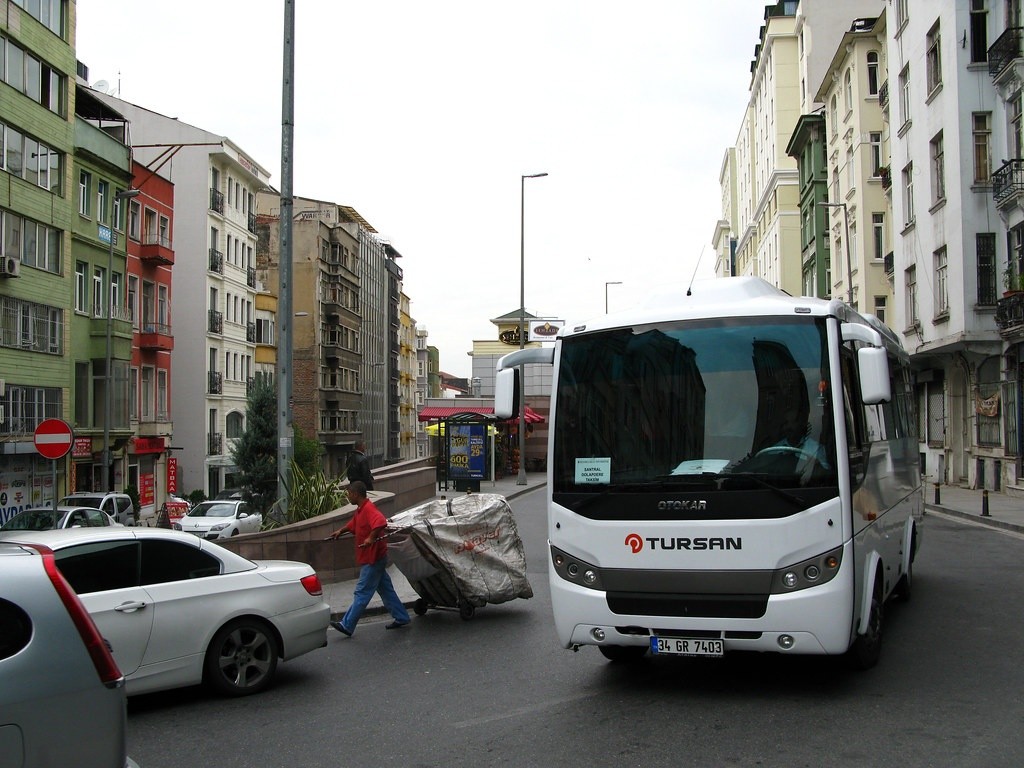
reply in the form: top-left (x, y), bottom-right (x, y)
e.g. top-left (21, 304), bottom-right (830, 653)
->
top-left (516, 173), bottom-right (551, 488)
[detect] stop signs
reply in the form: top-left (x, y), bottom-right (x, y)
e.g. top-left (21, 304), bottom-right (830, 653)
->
top-left (33, 419), bottom-right (73, 460)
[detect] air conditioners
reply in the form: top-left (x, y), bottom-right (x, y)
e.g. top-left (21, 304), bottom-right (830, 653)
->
top-left (0, 254), bottom-right (22, 279)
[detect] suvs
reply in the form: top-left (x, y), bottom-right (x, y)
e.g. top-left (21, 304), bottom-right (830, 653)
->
top-left (54, 489), bottom-right (135, 529)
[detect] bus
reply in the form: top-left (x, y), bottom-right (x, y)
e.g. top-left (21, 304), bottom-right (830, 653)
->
top-left (492, 274), bottom-right (929, 670)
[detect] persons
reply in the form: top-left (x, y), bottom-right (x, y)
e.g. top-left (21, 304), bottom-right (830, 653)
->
top-left (643, 391), bottom-right (687, 465)
top-left (328, 481), bottom-right (410, 638)
top-left (345, 439), bottom-right (374, 491)
top-left (768, 408), bottom-right (831, 470)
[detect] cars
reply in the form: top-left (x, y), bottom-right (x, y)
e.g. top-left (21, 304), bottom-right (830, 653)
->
top-left (172, 499), bottom-right (263, 541)
top-left (0, 524), bottom-right (329, 693)
top-left (0, 506), bottom-right (121, 542)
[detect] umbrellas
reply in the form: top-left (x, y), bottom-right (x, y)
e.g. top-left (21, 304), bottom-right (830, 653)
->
top-left (425, 421), bottom-right (499, 437)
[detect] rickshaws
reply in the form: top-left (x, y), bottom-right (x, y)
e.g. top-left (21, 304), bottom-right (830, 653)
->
top-left (323, 493), bottom-right (487, 619)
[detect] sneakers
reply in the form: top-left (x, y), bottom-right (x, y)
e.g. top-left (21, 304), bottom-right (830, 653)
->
top-left (385, 621), bottom-right (410, 629)
top-left (330, 622), bottom-right (351, 637)
